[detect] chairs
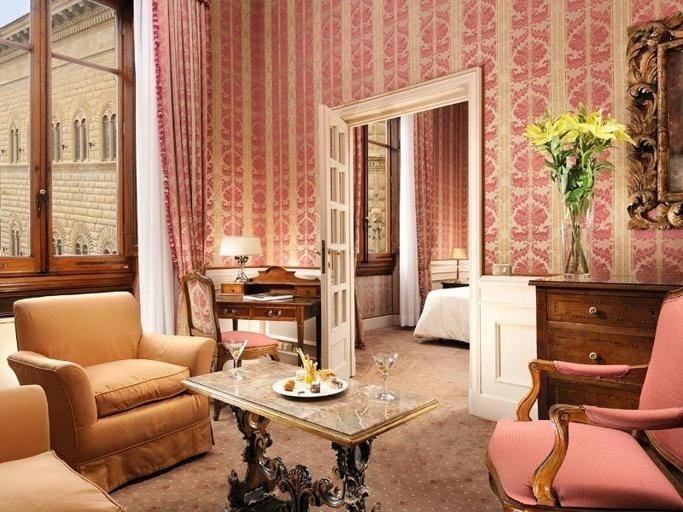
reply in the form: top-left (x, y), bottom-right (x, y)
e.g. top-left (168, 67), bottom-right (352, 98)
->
top-left (180, 269), bottom-right (280, 421)
top-left (0, 384), bottom-right (126, 512)
top-left (485, 287), bottom-right (683, 512)
top-left (7, 291), bottom-right (216, 462)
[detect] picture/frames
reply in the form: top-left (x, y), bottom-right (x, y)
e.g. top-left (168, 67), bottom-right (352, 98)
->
top-left (626, 9), bottom-right (682, 229)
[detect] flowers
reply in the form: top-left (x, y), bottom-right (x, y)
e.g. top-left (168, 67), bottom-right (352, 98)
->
top-left (522, 100), bottom-right (638, 275)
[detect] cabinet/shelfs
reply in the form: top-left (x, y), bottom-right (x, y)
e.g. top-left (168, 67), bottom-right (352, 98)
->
top-left (527, 281), bottom-right (683, 420)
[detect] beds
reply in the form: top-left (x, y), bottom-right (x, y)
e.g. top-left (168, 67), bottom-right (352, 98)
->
top-left (413, 286), bottom-right (470, 343)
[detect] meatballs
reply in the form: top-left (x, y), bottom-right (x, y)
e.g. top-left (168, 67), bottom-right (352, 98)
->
top-left (285, 380), bottom-right (295, 390)
top-left (311, 385), bottom-right (320, 393)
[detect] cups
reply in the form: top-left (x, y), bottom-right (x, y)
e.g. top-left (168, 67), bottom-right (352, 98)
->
top-left (492, 264), bottom-right (511, 275)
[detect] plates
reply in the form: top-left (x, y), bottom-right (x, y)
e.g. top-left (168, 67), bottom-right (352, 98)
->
top-left (271, 376), bottom-right (350, 398)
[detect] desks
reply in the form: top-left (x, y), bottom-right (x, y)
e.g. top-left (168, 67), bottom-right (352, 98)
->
top-left (215, 267), bottom-right (321, 369)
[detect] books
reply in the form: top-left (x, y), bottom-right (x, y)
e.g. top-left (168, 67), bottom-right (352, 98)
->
top-left (243, 292), bottom-right (293, 302)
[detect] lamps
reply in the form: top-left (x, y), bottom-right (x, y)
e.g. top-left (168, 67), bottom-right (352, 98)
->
top-left (448, 247), bottom-right (469, 280)
top-left (218, 236), bottom-right (263, 283)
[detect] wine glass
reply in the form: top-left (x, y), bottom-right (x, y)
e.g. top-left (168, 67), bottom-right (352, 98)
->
top-left (371, 352), bottom-right (401, 401)
top-left (222, 339), bottom-right (250, 382)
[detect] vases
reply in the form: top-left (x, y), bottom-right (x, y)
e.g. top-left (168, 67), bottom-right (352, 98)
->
top-left (550, 173), bottom-right (595, 282)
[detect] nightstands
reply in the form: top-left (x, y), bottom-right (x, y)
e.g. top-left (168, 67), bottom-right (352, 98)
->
top-left (441, 280), bottom-right (469, 288)
